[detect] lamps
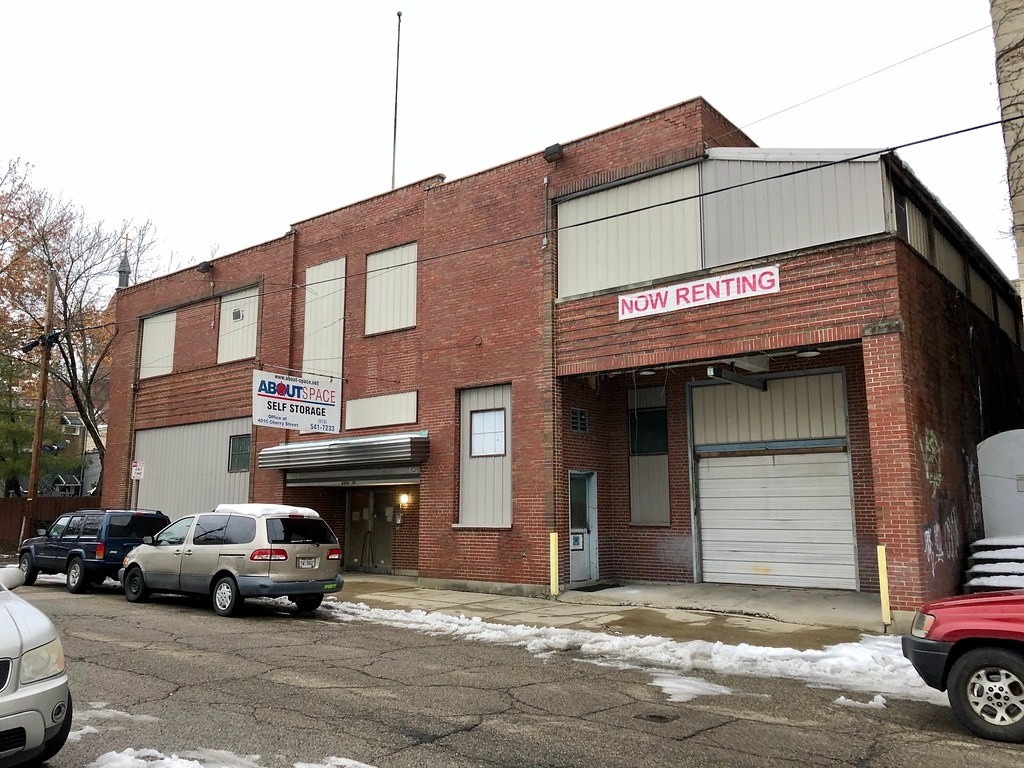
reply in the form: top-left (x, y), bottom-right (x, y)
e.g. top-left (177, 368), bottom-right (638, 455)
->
top-left (543, 144), bottom-right (563, 163)
top-left (636, 369), bottom-right (656, 376)
top-left (196, 262), bottom-right (214, 274)
top-left (795, 348), bottom-right (821, 357)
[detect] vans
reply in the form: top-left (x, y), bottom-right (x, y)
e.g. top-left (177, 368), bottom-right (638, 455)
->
top-left (117, 503), bottom-right (344, 616)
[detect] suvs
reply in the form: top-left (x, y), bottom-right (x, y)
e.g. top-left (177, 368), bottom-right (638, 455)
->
top-left (901, 588), bottom-right (1024, 743)
top-left (19, 507), bottom-right (171, 594)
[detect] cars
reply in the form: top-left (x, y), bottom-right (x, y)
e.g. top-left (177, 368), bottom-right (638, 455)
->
top-left (0, 580), bottom-right (73, 768)
